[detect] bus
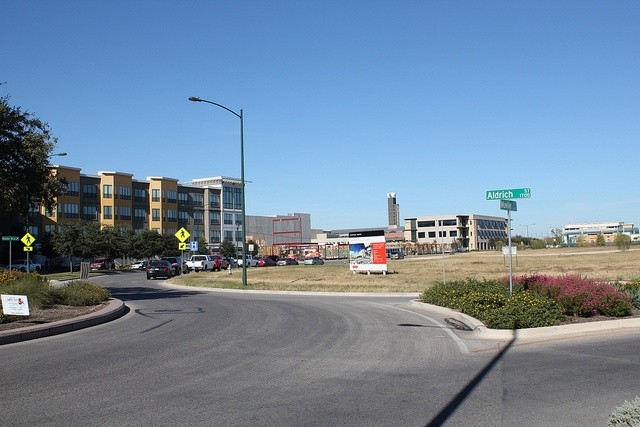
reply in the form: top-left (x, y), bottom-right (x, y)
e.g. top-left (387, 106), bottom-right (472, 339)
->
top-left (386, 248), bottom-right (402, 257)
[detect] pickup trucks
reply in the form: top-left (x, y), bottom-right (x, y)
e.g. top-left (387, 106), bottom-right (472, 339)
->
top-left (6, 259), bottom-right (41, 272)
top-left (186, 255), bottom-right (216, 272)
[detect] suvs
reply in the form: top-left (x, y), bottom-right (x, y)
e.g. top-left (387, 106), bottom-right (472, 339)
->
top-left (233, 254), bottom-right (259, 268)
top-left (161, 257), bottom-right (188, 275)
top-left (210, 254), bottom-right (229, 272)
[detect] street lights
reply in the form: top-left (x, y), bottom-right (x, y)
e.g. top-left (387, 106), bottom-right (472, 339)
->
top-left (236, 223), bottom-right (239, 268)
top-left (26, 152), bottom-right (68, 273)
top-left (189, 97), bottom-right (247, 286)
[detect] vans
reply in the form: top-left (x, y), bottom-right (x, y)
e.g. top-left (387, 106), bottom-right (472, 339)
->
top-left (391, 252), bottom-right (404, 260)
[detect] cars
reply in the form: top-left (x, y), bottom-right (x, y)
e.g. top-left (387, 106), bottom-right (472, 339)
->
top-left (259, 258), bottom-right (276, 266)
top-left (147, 261), bottom-right (175, 280)
top-left (304, 257), bottom-right (325, 265)
top-left (90, 258), bottom-right (115, 270)
top-left (143, 260), bottom-right (159, 269)
top-left (276, 258), bottom-right (299, 266)
top-left (286, 254), bottom-right (299, 260)
top-left (131, 261), bottom-right (145, 270)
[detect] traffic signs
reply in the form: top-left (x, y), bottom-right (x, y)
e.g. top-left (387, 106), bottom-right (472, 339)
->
top-left (486, 188), bottom-right (531, 200)
top-left (500, 200), bottom-right (518, 211)
top-left (2, 235), bottom-right (19, 240)
